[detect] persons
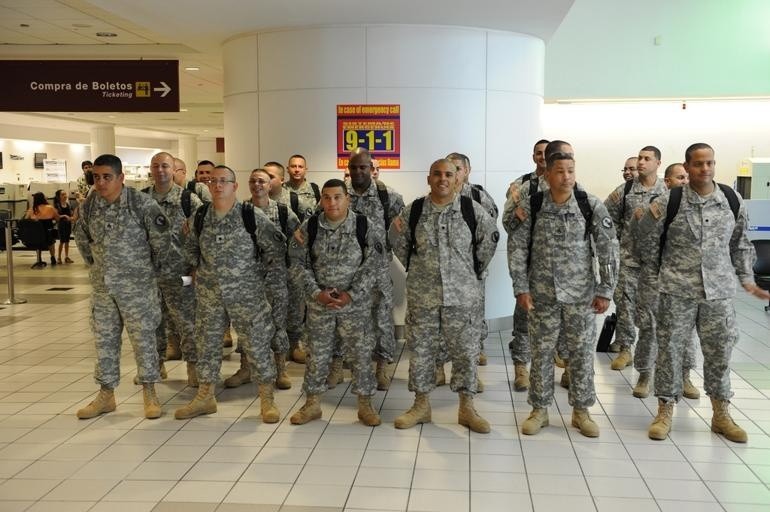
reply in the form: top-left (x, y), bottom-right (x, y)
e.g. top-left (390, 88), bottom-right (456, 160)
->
top-left (503, 140), bottom-right (770, 443)
top-left (52, 189), bottom-right (74, 264)
top-left (72, 147), bottom-right (500, 434)
top-left (24, 192), bottom-right (59, 266)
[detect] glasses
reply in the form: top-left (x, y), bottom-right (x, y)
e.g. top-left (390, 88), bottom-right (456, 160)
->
top-left (208, 179), bottom-right (236, 185)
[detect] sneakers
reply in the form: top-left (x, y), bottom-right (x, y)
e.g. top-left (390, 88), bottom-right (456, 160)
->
top-left (458, 393), bottom-right (490, 433)
top-left (258, 384), bottom-right (280, 424)
top-left (51, 256), bottom-right (73, 265)
top-left (173, 383), bottom-right (218, 419)
top-left (167, 338), bottom-right (183, 360)
top-left (75, 385), bottom-right (117, 418)
top-left (140, 383), bottom-right (162, 419)
top-left (394, 392), bottom-right (432, 429)
top-left (572, 406), bottom-right (600, 437)
top-left (184, 362), bottom-right (199, 387)
top-left (610, 349), bottom-right (701, 399)
top-left (290, 394), bottom-right (322, 424)
top-left (514, 350), bottom-right (568, 389)
top-left (522, 407), bottom-right (548, 435)
top-left (222, 323), bottom-right (395, 392)
top-left (648, 397), bottom-right (675, 441)
top-left (435, 340), bottom-right (487, 391)
top-left (357, 395), bottom-right (383, 427)
top-left (134, 362), bottom-right (169, 386)
top-left (709, 399), bottom-right (750, 443)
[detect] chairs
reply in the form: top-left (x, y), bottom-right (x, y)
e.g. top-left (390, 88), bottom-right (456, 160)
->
top-left (10, 217), bottom-right (61, 271)
